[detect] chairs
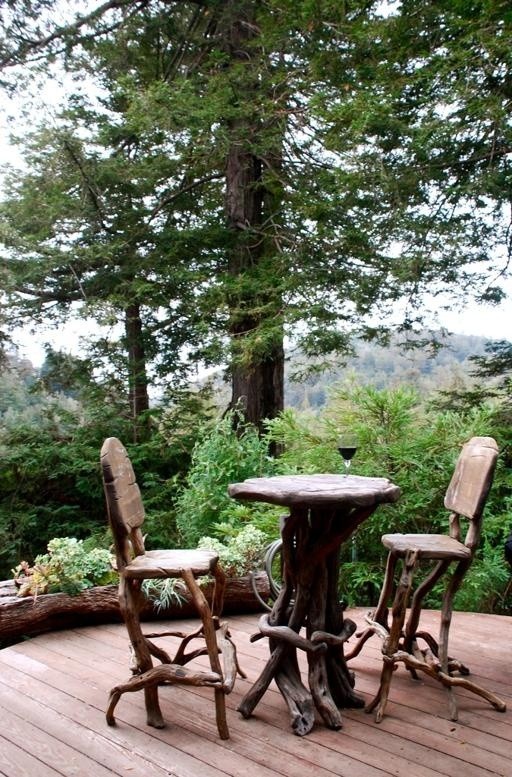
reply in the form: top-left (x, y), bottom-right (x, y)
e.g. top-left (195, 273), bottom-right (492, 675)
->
top-left (345, 435), bottom-right (505, 723)
top-left (99, 435), bottom-right (246, 739)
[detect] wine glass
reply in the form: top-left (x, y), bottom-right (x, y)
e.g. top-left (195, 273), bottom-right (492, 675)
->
top-left (338, 433), bottom-right (358, 480)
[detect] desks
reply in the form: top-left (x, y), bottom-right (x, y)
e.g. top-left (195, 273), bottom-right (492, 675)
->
top-left (227, 474), bottom-right (404, 738)
